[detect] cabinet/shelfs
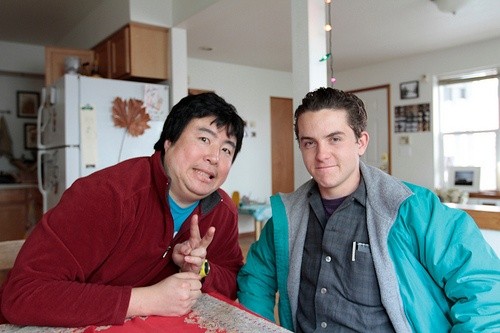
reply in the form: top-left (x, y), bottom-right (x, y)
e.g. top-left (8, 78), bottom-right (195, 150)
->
top-left (0, 187), bottom-right (43, 242)
top-left (45, 21), bottom-right (170, 84)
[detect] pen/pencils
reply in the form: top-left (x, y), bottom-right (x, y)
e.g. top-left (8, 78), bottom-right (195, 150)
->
top-left (352, 241), bottom-right (356, 261)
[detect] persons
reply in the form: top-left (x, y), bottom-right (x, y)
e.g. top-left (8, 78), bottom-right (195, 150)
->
top-left (0, 92), bottom-right (246, 327)
top-left (237, 87), bottom-right (500, 333)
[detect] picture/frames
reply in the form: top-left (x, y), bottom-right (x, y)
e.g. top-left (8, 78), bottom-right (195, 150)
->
top-left (399, 81), bottom-right (419, 99)
top-left (24, 123), bottom-right (38, 149)
top-left (16, 90), bottom-right (40, 119)
top-left (447, 167), bottom-right (481, 193)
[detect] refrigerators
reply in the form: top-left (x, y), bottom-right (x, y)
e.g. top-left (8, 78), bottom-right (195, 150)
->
top-left (37, 74), bottom-right (170, 214)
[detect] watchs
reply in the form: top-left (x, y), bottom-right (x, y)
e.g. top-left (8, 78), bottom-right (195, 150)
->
top-left (198, 258), bottom-right (211, 279)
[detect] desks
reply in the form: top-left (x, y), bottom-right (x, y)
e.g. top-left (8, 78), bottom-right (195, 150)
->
top-left (0, 290), bottom-right (291, 333)
top-left (237, 199), bottom-right (272, 242)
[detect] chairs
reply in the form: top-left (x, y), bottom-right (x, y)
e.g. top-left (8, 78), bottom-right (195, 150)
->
top-left (0, 240), bottom-right (28, 271)
